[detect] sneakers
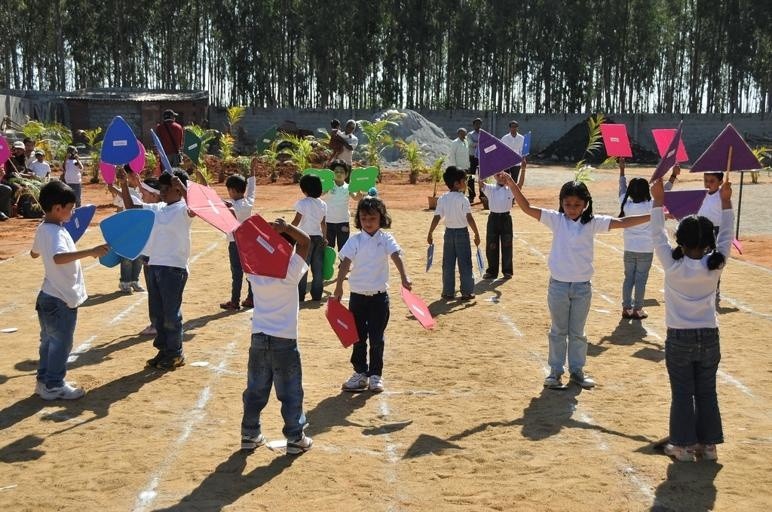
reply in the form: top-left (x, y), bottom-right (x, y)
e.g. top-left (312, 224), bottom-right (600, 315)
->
top-left (242, 298), bottom-right (254, 307)
top-left (504, 273), bottom-right (512, 279)
top-left (369, 375), bottom-right (384, 392)
top-left (241, 433), bottom-right (266, 450)
top-left (483, 273), bottom-right (497, 279)
top-left (544, 373), bottom-right (561, 386)
top-left (697, 440), bottom-right (717, 460)
top-left (146, 353), bottom-right (185, 371)
top-left (463, 293), bottom-right (475, 300)
top-left (118, 278), bottom-right (145, 294)
top-left (288, 435), bottom-right (313, 454)
top-left (570, 371), bottom-right (596, 387)
top-left (665, 443), bottom-right (695, 462)
top-left (220, 302), bottom-right (240, 309)
top-left (622, 307), bottom-right (648, 319)
top-left (35, 379), bottom-right (85, 401)
top-left (342, 373), bottom-right (369, 389)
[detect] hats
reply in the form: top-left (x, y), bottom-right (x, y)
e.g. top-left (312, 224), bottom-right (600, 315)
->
top-left (163, 109), bottom-right (179, 119)
top-left (35, 150), bottom-right (45, 156)
top-left (13, 141), bottom-right (25, 151)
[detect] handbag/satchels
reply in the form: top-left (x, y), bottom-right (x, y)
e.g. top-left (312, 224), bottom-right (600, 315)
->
top-left (177, 151), bottom-right (184, 163)
